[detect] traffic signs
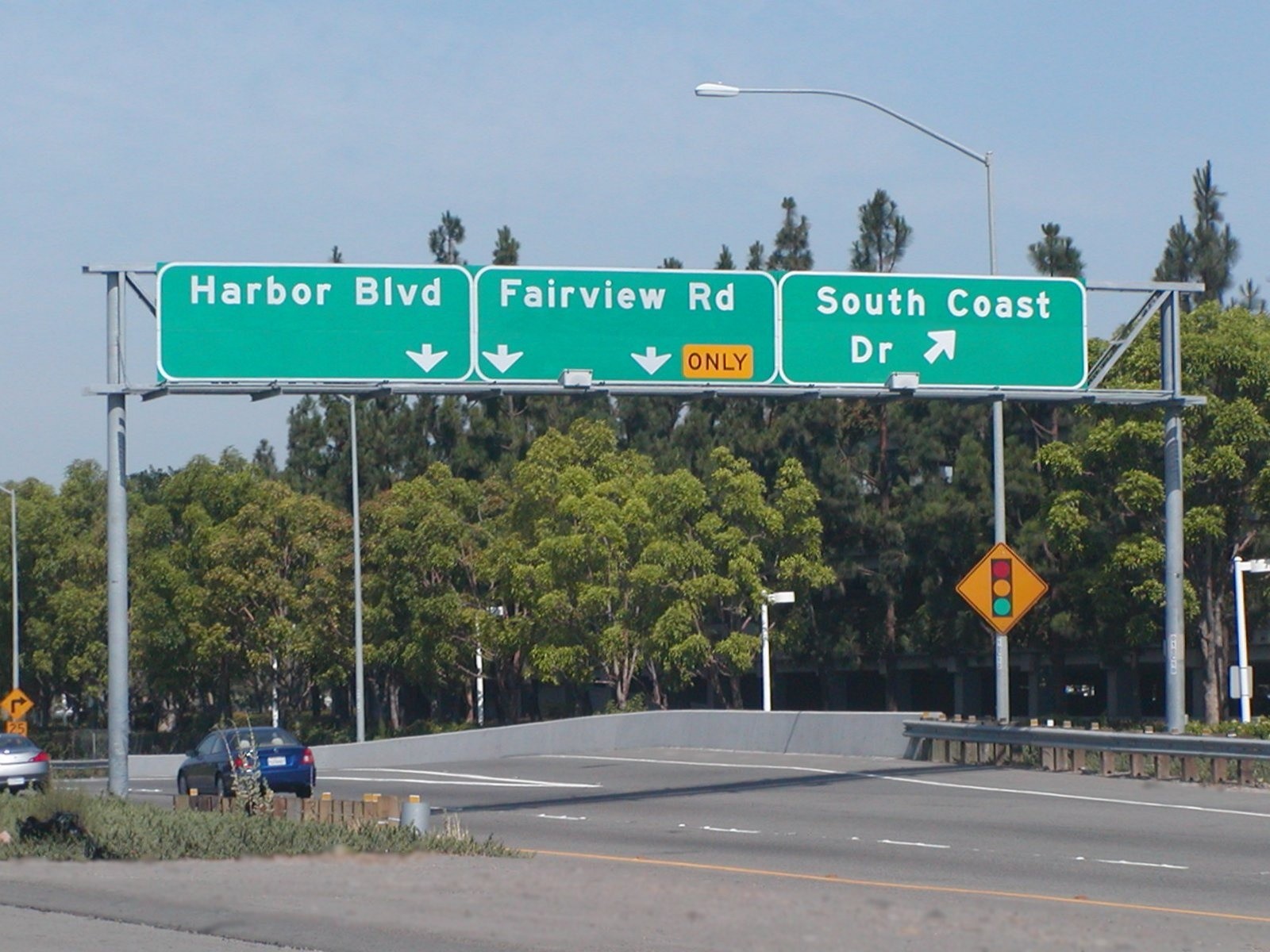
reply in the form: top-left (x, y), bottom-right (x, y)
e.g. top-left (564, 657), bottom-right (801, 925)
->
top-left (779, 270), bottom-right (1088, 390)
top-left (156, 262), bottom-right (474, 382)
top-left (475, 267), bottom-right (777, 387)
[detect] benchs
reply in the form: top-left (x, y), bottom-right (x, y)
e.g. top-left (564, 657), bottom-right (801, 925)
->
top-left (239, 738), bottom-right (284, 748)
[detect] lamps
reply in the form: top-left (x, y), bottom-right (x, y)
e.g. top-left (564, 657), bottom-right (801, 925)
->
top-left (885, 372), bottom-right (921, 392)
top-left (558, 369), bottom-right (594, 389)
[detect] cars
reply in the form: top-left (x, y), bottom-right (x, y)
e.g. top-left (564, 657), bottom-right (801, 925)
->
top-left (177, 727), bottom-right (316, 803)
top-left (0, 733), bottom-right (52, 796)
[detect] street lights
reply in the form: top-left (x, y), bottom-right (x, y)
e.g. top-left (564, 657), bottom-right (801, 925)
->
top-left (1235, 555), bottom-right (1270, 727)
top-left (475, 607), bottom-right (505, 727)
top-left (760, 589), bottom-right (795, 711)
top-left (696, 83), bottom-right (1009, 763)
